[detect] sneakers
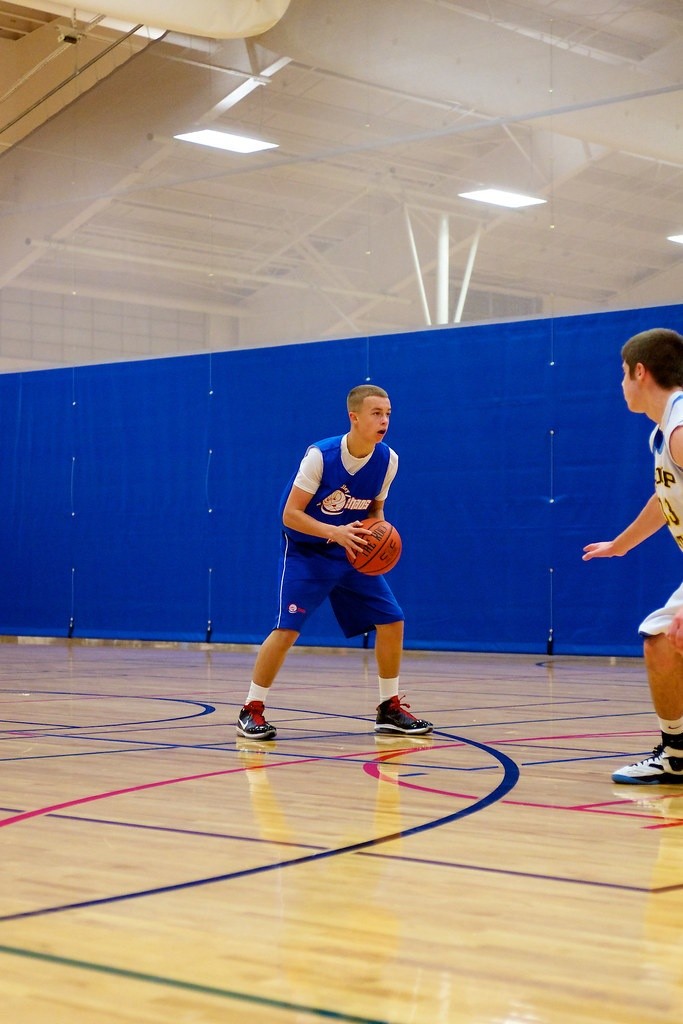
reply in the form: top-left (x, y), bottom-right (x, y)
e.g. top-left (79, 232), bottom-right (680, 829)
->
top-left (612, 743), bottom-right (683, 784)
top-left (235, 700), bottom-right (278, 740)
top-left (373, 694), bottom-right (433, 734)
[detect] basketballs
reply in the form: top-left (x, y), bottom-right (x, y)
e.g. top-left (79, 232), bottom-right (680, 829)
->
top-left (345, 517), bottom-right (403, 577)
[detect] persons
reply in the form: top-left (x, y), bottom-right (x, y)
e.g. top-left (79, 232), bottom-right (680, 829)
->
top-left (235, 384), bottom-right (435, 739)
top-left (580, 327), bottom-right (683, 787)
